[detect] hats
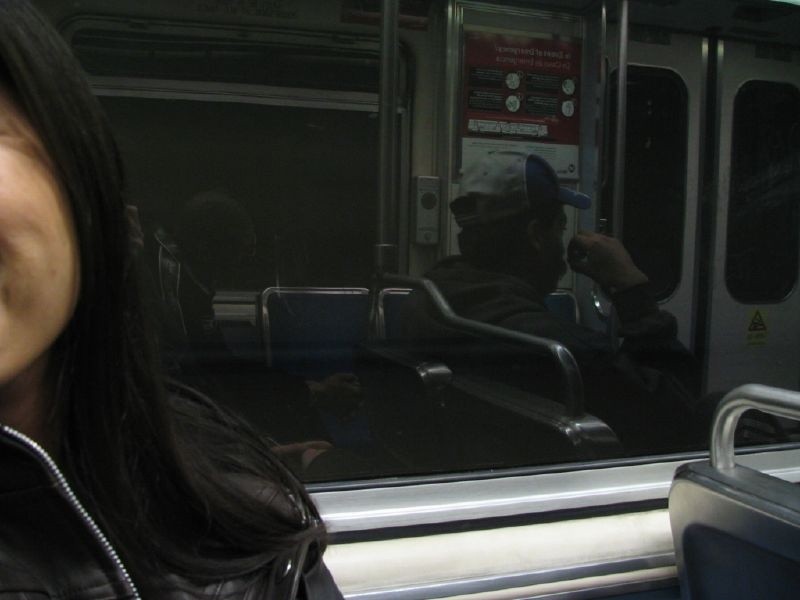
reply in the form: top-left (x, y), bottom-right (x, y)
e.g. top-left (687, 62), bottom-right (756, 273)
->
top-left (447, 149), bottom-right (593, 227)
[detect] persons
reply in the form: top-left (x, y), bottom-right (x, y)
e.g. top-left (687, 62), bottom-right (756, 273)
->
top-left (121, 202), bottom-right (232, 410)
top-left (387, 149), bottom-right (705, 438)
top-left (0, 0), bottom-right (344, 600)
top-left (211, 284), bottom-right (419, 481)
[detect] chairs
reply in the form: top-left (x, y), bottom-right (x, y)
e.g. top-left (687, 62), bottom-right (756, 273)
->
top-left (664, 377), bottom-right (800, 600)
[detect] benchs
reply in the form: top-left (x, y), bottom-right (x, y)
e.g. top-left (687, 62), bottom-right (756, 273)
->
top-left (242, 268), bottom-right (624, 471)
